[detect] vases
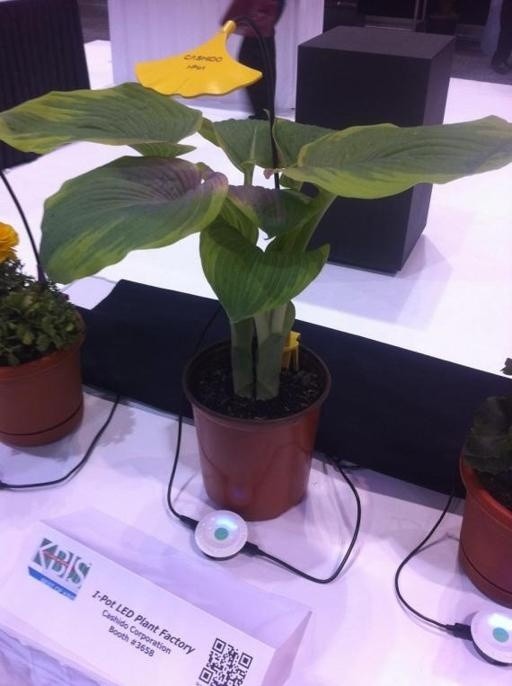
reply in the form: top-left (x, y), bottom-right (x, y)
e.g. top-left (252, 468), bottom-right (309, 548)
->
top-left (0, 314), bottom-right (86, 449)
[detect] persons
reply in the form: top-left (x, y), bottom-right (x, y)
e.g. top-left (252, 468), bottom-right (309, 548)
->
top-left (220, 1), bottom-right (286, 119)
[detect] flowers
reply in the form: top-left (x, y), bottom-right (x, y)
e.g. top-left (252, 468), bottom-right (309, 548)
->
top-left (0, 222), bottom-right (81, 364)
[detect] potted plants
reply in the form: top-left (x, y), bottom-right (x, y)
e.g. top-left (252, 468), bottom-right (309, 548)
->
top-left (1, 82), bottom-right (510, 520)
top-left (458, 357), bottom-right (510, 611)
